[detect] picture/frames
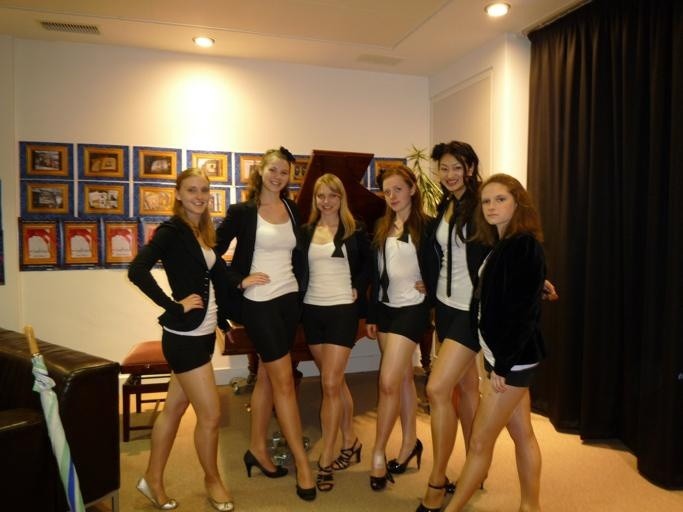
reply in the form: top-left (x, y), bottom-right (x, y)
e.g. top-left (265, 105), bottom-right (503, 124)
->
top-left (17, 140), bottom-right (264, 272)
top-left (360, 157), bottom-right (407, 200)
top-left (288, 154), bottom-right (312, 200)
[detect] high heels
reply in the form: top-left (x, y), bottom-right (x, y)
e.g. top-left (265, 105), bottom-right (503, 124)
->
top-left (417, 475), bottom-right (448, 512)
top-left (317, 460), bottom-right (333, 491)
top-left (387, 439), bottom-right (422, 473)
top-left (296, 465), bottom-right (316, 500)
top-left (448, 472), bottom-right (487, 494)
top-left (333, 438), bottom-right (362, 470)
top-left (136, 477), bottom-right (177, 509)
top-left (370, 454), bottom-right (388, 490)
top-left (204, 477), bottom-right (234, 512)
top-left (244, 450), bottom-right (288, 477)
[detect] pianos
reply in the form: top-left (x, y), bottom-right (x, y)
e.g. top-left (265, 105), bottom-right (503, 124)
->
top-left (214, 150), bottom-right (433, 401)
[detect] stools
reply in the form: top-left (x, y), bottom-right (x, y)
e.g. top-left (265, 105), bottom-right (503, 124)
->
top-left (115, 338), bottom-right (174, 443)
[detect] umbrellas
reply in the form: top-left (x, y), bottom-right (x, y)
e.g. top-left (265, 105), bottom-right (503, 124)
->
top-left (23, 324), bottom-right (85, 512)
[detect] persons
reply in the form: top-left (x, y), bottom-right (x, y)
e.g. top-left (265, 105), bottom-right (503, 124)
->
top-left (297, 172), bottom-right (367, 494)
top-left (126, 169), bottom-right (234, 512)
top-left (443, 172), bottom-right (543, 511)
top-left (214, 144), bottom-right (318, 502)
top-left (415, 139), bottom-right (559, 512)
top-left (362, 164), bottom-right (437, 490)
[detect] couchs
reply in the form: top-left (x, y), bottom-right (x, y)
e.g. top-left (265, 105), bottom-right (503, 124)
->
top-left (1, 327), bottom-right (122, 512)
